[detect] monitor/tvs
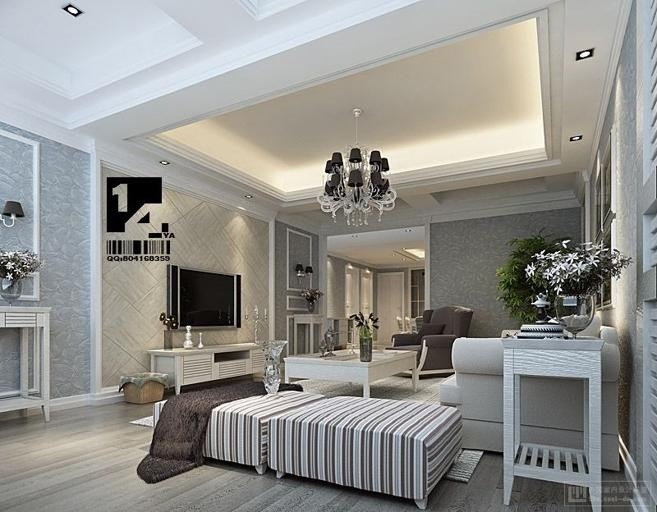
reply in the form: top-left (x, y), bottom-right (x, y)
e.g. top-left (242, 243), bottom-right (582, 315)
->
top-left (178, 267), bottom-right (236, 328)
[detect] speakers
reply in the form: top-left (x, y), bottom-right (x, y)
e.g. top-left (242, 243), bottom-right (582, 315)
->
top-left (166, 263), bottom-right (178, 330)
top-left (235, 274), bottom-right (241, 328)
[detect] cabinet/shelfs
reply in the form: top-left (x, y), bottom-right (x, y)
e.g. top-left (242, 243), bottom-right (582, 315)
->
top-left (0, 304), bottom-right (53, 422)
top-left (293, 314), bottom-right (324, 354)
top-left (148, 341), bottom-right (265, 396)
top-left (500, 335), bottom-right (607, 511)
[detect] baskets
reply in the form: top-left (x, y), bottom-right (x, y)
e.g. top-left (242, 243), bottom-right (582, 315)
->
top-left (124, 376), bottom-right (166, 404)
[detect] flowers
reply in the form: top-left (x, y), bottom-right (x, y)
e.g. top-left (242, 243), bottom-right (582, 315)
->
top-left (524, 238), bottom-right (631, 293)
top-left (1, 249), bottom-right (45, 287)
top-left (301, 287), bottom-right (322, 301)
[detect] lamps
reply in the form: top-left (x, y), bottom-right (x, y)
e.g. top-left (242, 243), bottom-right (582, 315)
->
top-left (0, 201), bottom-right (24, 227)
top-left (316, 108), bottom-right (398, 224)
top-left (296, 263), bottom-right (313, 279)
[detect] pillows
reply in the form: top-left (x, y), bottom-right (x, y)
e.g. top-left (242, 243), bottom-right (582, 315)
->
top-left (417, 321), bottom-right (447, 346)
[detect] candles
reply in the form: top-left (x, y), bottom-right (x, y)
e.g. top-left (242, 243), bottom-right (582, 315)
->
top-left (244, 306), bottom-right (268, 317)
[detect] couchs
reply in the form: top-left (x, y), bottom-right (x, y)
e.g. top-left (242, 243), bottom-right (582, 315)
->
top-left (153, 388), bottom-right (326, 477)
top-left (388, 305), bottom-right (473, 378)
top-left (265, 396), bottom-right (466, 512)
top-left (439, 314), bottom-right (621, 474)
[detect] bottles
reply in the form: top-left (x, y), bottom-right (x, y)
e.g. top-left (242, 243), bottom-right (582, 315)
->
top-left (183, 325), bottom-right (193, 349)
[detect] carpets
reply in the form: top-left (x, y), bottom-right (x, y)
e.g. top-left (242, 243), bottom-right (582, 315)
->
top-left (129, 370), bottom-right (483, 486)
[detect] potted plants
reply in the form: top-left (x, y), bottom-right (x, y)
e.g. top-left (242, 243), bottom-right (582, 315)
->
top-left (347, 312), bottom-right (379, 362)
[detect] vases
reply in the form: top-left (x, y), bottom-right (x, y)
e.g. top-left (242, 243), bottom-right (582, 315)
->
top-left (1, 278), bottom-right (24, 306)
top-left (308, 302), bottom-right (315, 312)
top-left (554, 294), bottom-right (597, 338)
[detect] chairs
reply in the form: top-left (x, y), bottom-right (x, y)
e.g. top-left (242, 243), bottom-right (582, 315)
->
top-left (396, 314), bottom-right (416, 333)
